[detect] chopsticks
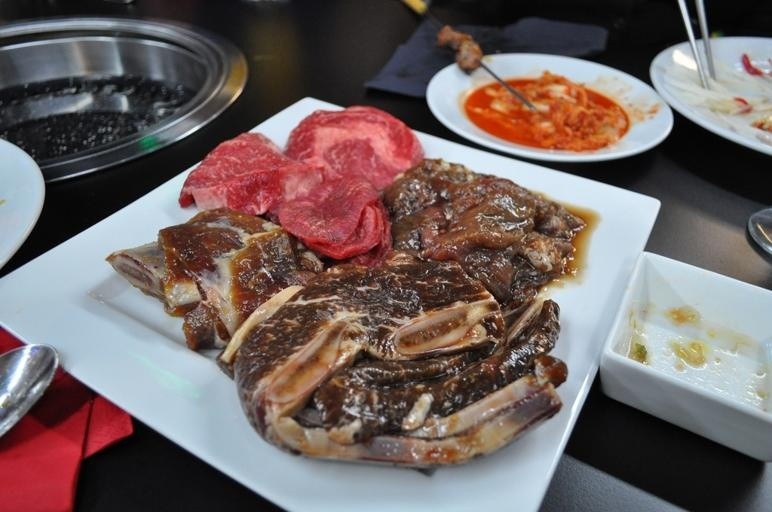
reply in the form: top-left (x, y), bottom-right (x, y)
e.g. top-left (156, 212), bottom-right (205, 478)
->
top-left (675, 0), bottom-right (714, 89)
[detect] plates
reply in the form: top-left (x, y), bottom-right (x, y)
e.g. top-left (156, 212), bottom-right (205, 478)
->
top-left (0, 134), bottom-right (48, 273)
top-left (0, 94), bottom-right (663, 512)
top-left (0, 16), bottom-right (248, 190)
top-left (426, 51), bottom-right (676, 161)
top-left (650, 38), bottom-right (771, 157)
top-left (602, 251), bottom-right (771, 462)
